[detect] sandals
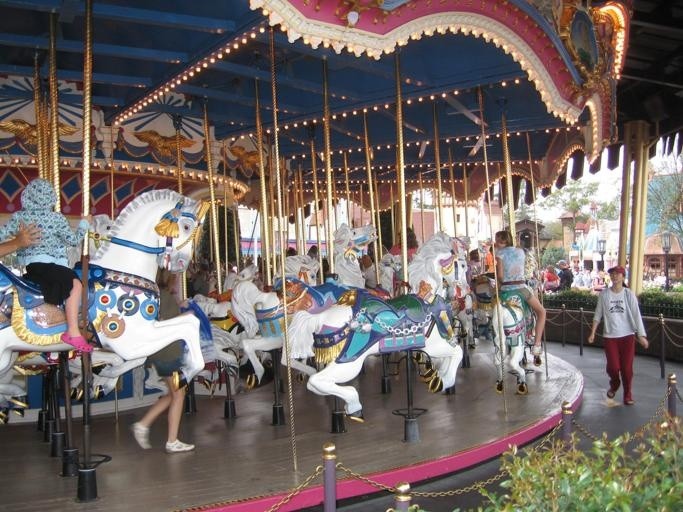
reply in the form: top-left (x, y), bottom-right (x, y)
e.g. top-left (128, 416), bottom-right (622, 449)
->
top-left (62, 333), bottom-right (92, 353)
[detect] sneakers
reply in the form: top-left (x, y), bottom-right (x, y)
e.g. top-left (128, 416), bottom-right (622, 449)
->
top-left (166, 440), bottom-right (194, 452)
top-left (532, 346), bottom-right (542, 352)
top-left (133, 422), bottom-right (152, 449)
top-left (625, 400), bottom-right (634, 405)
top-left (608, 386), bottom-right (616, 398)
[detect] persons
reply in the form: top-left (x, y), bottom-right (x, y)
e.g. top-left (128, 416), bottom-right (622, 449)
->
top-left (389, 227), bottom-right (418, 262)
top-left (0, 178), bottom-right (94, 352)
top-left (283, 247), bottom-right (297, 258)
top-left (493, 231), bottom-right (546, 357)
top-left (130, 268), bottom-right (195, 454)
top-left (591, 270), bottom-right (649, 404)
top-left (0, 219), bottom-right (44, 258)
top-left (529, 259), bottom-right (631, 292)
top-left (484, 247), bottom-right (496, 273)
top-left (654, 270), bottom-right (667, 287)
top-left (464, 249), bottom-right (485, 286)
top-left (308, 247), bottom-right (331, 280)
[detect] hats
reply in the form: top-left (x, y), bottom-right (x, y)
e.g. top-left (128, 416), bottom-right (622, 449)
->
top-left (608, 266), bottom-right (625, 276)
top-left (556, 260), bottom-right (566, 265)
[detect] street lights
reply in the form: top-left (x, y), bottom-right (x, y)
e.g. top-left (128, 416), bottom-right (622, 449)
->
top-left (660, 230), bottom-right (671, 291)
top-left (598, 238), bottom-right (607, 271)
